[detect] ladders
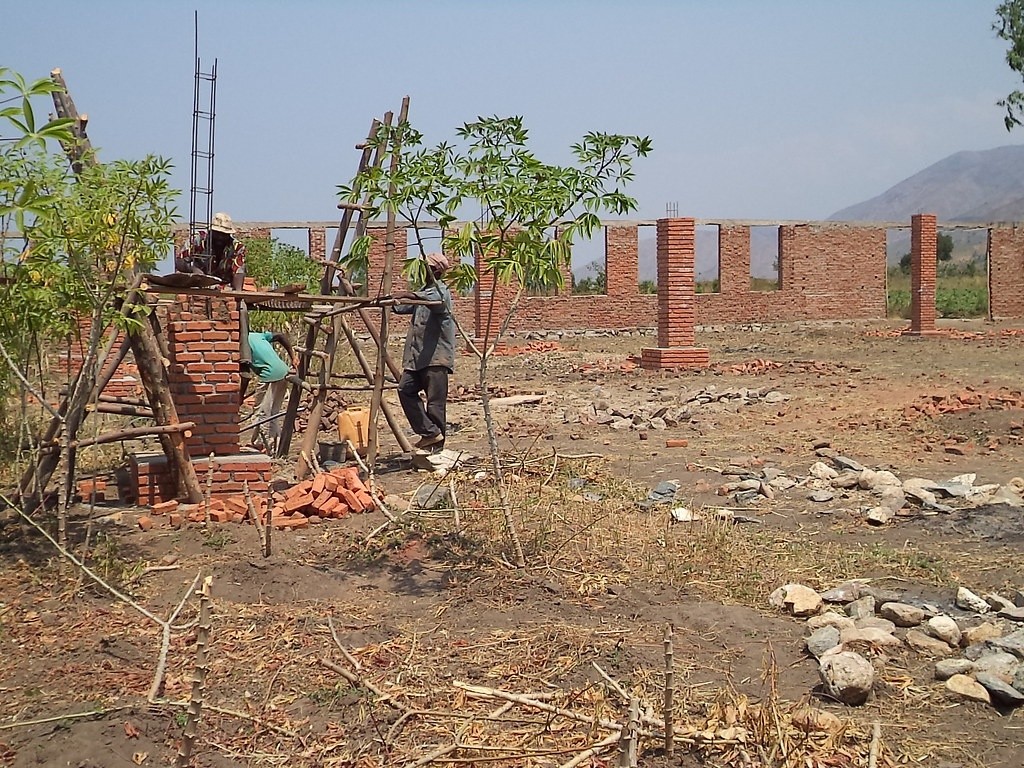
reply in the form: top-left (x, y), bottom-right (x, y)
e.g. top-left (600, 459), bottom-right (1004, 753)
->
top-left (279, 110), bottom-right (429, 476)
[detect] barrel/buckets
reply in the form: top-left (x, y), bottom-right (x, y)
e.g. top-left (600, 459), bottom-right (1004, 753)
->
top-left (317, 440), bottom-right (348, 463)
top-left (338, 406), bottom-right (380, 460)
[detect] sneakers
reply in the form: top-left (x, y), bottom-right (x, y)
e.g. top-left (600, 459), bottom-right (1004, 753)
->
top-left (414, 432), bottom-right (445, 452)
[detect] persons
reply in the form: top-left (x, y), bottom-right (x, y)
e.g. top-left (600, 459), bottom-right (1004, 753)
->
top-left (177, 214), bottom-right (247, 291)
top-left (390, 252), bottom-right (455, 454)
top-left (240, 332), bottom-right (301, 450)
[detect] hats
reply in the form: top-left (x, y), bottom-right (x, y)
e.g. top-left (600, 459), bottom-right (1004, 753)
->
top-left (419, 253), bottom-right (448, 272)
top-left (207, 213), bottom-right (236, 234)
